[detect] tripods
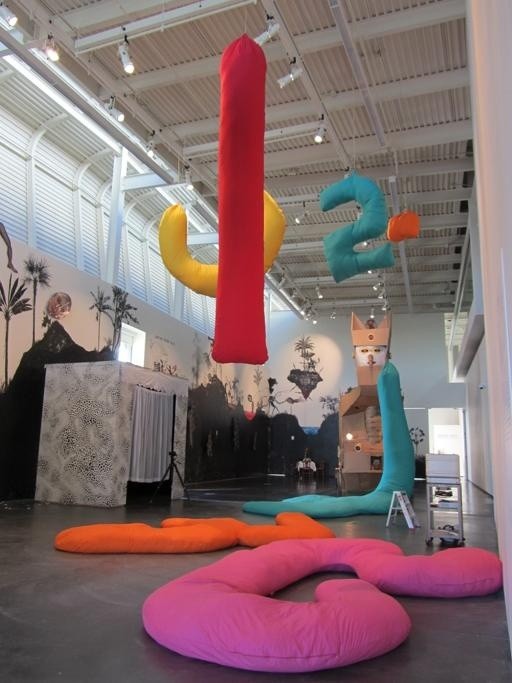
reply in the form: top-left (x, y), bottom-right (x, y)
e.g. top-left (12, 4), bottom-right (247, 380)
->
top-left (150, 451), bottom-right (190, 502)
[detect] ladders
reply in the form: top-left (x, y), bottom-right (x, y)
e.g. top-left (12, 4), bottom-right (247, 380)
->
top-left (385, 491), bottom-right (421, 530)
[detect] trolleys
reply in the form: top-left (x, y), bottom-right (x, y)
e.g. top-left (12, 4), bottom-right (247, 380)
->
top-left (424, 452), bottom-right (466, 546)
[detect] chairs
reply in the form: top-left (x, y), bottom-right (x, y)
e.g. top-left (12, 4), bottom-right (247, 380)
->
top-left (292, 461), bottom-right (328, 480)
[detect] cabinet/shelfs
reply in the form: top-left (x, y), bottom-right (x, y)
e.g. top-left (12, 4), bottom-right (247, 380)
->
top-left (425, 482), bottom-right (465, 545)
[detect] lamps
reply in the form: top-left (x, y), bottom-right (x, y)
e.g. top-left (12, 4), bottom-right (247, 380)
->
top-left (109, 27), bottom-right (143, 75)
top-left (251, 13), bottom-right (392, 313)
top-left (3, 8), bottom-right (319, 327)
